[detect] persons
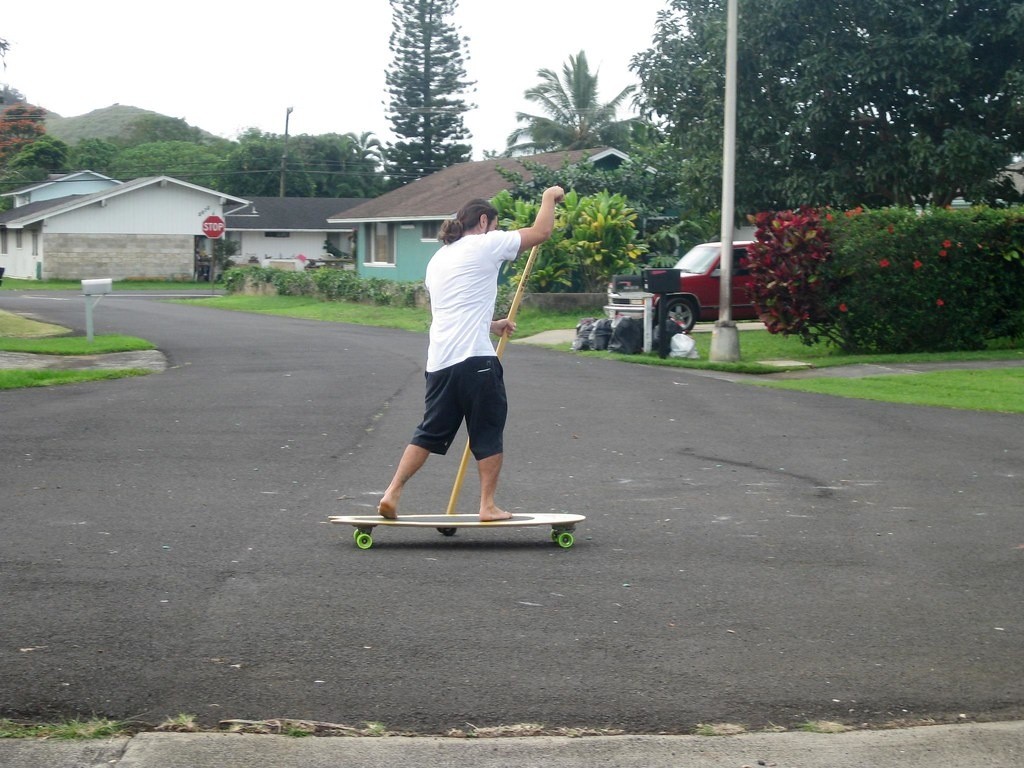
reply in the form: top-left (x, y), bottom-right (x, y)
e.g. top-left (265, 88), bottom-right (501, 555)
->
top-left (379, 186), bottom-right (565, 519)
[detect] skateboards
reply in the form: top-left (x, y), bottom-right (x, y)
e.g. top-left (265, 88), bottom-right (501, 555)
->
top-left (326, 511), bottom-right (587, 549)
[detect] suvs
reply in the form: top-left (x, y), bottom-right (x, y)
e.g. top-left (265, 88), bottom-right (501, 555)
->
top-left (604, 241), bottom-right (764, 332)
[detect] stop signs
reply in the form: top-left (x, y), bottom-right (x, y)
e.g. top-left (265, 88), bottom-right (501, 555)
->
top-left (202, 216), bottom-right (225, 239)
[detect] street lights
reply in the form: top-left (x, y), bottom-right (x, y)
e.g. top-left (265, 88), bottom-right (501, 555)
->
top-left (280, 106), bottom-right (294, 196)
